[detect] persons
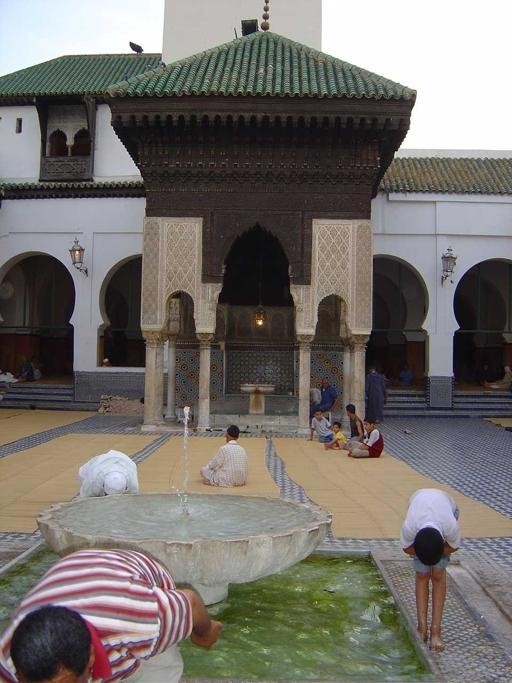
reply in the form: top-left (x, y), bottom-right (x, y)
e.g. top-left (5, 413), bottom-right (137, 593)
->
top-left (399, 364), bottom-right (414, 386)
top-left (198, 425), bottom-right (250, 488)
top-left (366, 361), bottom-right (388, 422)
top-left (14, 356), bottom-right (42, 382)
top-left (0, 548), bottom-right (223, 683)
top-left (308, 378), bottom-right (383, 459)
top-left (400, 488), bottom-right (460, 653)
top-left (477, 362), bottom-right (512, 389)
top-left (79, 450), bottom-right (141, 498)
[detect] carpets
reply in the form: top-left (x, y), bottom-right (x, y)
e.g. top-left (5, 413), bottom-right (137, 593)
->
top-left (136, 436), bottom-right (279, 499)
top-left (1, 432), bottom-right (163, 535)
top-left (1, 409), bottom-right (99, 448)
top-left (484, 418), bottom-right (511, 428)
top-left (270, 437), bottom-right (511, 540)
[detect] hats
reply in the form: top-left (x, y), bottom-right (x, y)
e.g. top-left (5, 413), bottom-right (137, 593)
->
top-left (103, 472), bottom-right (126, 495)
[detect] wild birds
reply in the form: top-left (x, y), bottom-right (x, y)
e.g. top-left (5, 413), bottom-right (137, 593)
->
top-left (128, 40), bottom-right (144, 55)
